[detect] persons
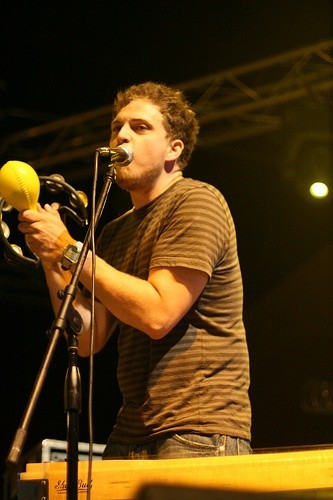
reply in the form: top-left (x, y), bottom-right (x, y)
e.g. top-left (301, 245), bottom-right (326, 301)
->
top-left (18, 81), bottom-right (253, 461)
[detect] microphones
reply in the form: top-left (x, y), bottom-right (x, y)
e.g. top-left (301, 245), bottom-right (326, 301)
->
top-left (97, 144), bottom-right (134, 166)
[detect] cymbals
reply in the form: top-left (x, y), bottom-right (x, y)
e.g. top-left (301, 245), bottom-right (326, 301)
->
top-left (0, 172), bottom-right (92, 272)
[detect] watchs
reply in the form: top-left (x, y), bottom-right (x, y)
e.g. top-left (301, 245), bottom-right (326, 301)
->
top-left (61, 241), bottom-right (84, 271)
